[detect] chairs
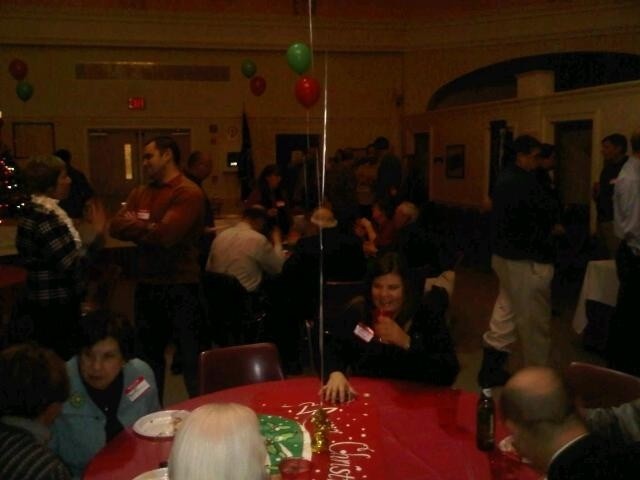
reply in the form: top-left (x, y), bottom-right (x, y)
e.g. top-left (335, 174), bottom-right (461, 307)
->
top-left (564, 361), bottom-right (639, 415)
top-left (191, 340), bottom-right (290, 397)
top-left (195, 270), bottom-right (250, 322)
top-left (302, 277), bottom-right (365, 377)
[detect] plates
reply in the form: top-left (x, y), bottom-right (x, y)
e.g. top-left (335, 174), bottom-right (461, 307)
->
top-left (132, 409), bottom-right (190, 438)
top-left (498, 435), bottom-right (533, 465)
top-left (131, 466), bottom-right (169, 480)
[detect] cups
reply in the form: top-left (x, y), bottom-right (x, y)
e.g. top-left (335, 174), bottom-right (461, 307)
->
top-left (288, 231), bottom-right (296, 250)
top-left (278, 457), bottom-right (314, 479)
top-left (368, 308), bottom-right (394, 341)
top-left (135, 208), bottom-right (151, 221)
top-left (488, 450), bottom-right (523, 479)
top-left (431, 388), bottom-right (461, 428)
top-left (295, 214), bottom-right (305, 236)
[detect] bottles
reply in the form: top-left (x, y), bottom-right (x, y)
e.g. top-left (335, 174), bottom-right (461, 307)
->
top-left (475, 385), bottom-right (496, 450)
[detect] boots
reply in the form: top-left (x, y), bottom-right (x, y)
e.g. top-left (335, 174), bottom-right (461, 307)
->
top-left (477, 342), bottom-right (510, 388)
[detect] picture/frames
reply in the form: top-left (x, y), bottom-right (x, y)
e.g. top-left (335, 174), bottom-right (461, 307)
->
top-left (444, 143), bottom-right (468, 180)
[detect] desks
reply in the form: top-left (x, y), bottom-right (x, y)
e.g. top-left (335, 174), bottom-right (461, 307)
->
top-left (78, 373), bottom-right (550, 480)
top-left (573, 261), bottom-right (622, 355)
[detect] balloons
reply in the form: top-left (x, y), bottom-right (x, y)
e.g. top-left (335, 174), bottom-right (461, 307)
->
top-left (286, 40), bottom-right (311, 74)
top-left (241, 58), bottom-right (258, 80)
top-left (15, 80), bottom-right (33, 102)
top-left (251, 77), bottom-right (267, 98)
top-left (10, 58), bottom-right (29, 80)
top-left (293, 76), bottom-right (320, 108)
